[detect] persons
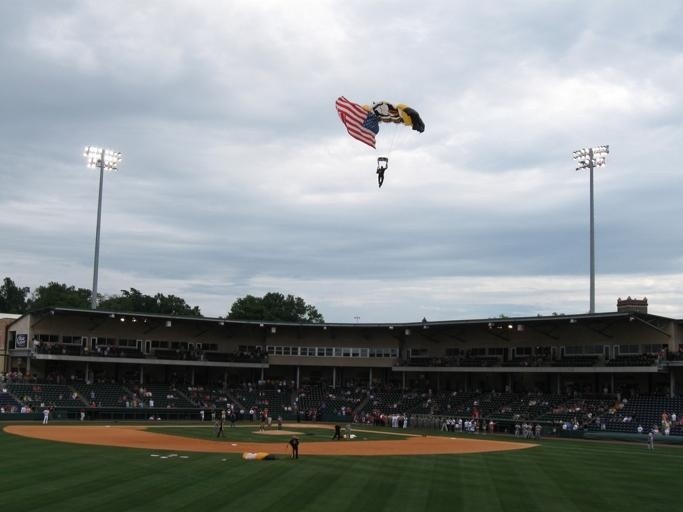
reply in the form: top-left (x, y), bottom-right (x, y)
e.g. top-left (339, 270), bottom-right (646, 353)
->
top-left (288, 434), bottom-right (298, 458)
top-left (1, 362), bottom-right (682, 440)
top-left (646, 428), bottom-right (656, 450)
top-left (375, 162), bottom-right (387, 187)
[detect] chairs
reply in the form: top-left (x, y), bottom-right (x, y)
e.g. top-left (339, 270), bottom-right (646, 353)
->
top-left (0, 381), bottom-right (683, 438)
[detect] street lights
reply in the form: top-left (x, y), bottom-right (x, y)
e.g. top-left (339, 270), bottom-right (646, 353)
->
top-left (82, 144), bottom-right (122, 310)
top-left (572, 145), bottom-right (609, 313)
